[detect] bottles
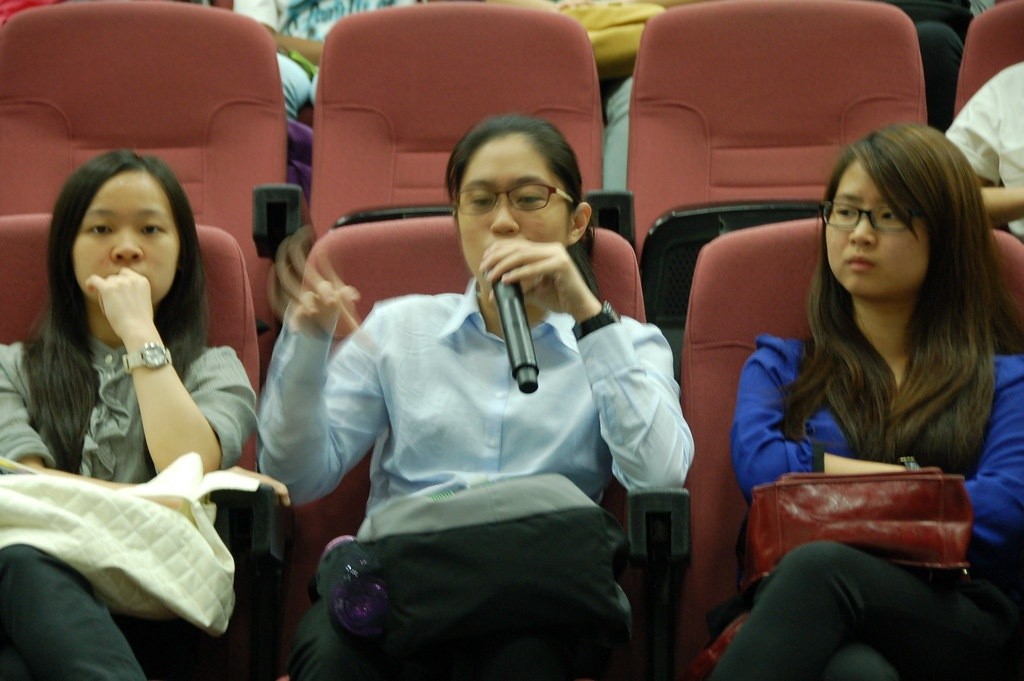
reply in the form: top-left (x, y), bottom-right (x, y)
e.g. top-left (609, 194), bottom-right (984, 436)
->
top-left (319, 533), bottom-right (388, 640)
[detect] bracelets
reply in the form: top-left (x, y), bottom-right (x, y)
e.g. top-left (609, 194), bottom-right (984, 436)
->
top-left (897, 453), bottom-right (921, 472)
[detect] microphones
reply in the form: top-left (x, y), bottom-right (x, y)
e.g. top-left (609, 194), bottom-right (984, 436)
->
top-left (483, 263), bottom-right (541, 395)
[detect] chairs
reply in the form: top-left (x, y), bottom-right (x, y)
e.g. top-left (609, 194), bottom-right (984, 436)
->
top-left (0, 209), bottom-right (296, 680)
top-left (0, 0), bottom-right (291, 388)
top-left (305, 214), bottom-right (695, 680)
top-left (625, 2), bottom-right (927, 377)
top-left (681, 215), bottom-right (1024, 681)
top-left (310, 0), bottom-right (640, 264)
top-left (954, 0), bottom-right (1024, 128)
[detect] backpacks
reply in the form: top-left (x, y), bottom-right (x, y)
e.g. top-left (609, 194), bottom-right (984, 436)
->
top-left (316, 470), bottom-right (636, 642)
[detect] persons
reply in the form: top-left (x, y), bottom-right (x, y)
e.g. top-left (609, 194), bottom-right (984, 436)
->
top-left (0, 147), bottom-right (260, 681)
top-left (229, 0), bottom-right (433, 123)
top-left (704, 123), bottom-right (1024, 679)
top-left (946, 60), bottom-right (1024, 242)
top-left (256, 111), bottom-right (693, 681)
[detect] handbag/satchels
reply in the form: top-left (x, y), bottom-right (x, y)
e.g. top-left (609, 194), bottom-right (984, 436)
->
top-left (739, 467), bottom-right (973, 583)
top-left (0, 452), bottom-right (263, 641)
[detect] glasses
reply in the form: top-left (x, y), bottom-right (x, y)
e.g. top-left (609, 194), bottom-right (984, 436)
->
top-left (456, 183), bottom-right (574, 214)
top-left (820, 199), bottom-right (925, 230)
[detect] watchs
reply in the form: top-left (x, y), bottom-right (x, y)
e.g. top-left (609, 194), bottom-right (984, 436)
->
top-left (571, 299), bottom-right (620, 342)
top-left (122, 344), bottom-right (173, 374)
